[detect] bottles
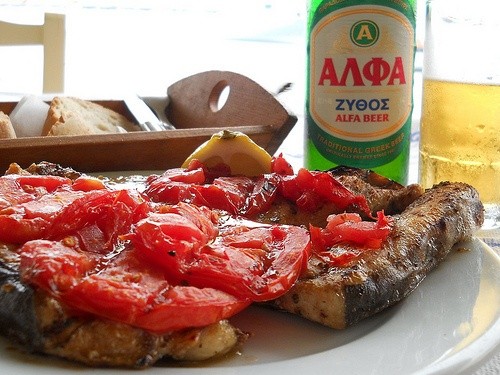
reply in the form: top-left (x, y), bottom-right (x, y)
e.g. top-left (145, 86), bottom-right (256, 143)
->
top-left (303, 0), bottom-right (419, 190)
top-left (411, 1), bottom-right (500, 233)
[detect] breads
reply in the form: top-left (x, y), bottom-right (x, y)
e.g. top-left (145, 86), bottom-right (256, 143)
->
top-left (41, 96), bottom-right (140, 136)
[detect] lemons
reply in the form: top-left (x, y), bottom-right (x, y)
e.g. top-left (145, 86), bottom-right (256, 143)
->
top-left (181, 129), bottom-right (272, 176)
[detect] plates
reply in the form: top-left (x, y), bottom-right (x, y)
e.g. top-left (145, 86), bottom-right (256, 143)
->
top-left (0, 166), bottom-right (500, 375)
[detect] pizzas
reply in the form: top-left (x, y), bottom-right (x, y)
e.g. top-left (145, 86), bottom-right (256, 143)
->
top-left (0, 154), bottom-right (484, 371)
